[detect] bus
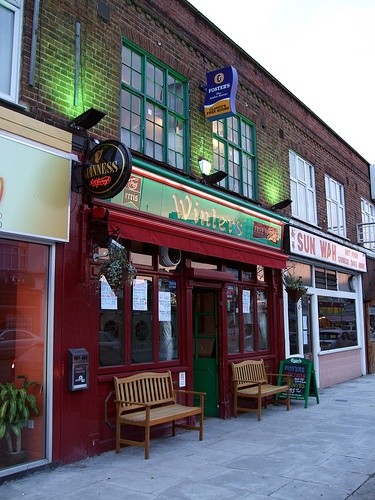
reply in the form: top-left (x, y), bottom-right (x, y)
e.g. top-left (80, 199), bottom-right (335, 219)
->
top-left (329, 319), bottom-right (355, 329)
top-left (318, 301), bottom-right (345, 314)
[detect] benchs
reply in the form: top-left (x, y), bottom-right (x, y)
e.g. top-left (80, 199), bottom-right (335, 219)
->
top-left (114, 370), bottom-right (206, 459)
top-left (231, 359), bottom-right (292, 421)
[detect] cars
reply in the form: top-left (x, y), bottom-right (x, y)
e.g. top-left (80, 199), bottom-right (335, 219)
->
top-left (319, 327), bottom-right (342, 349)
top-left (11, 343), bottom-right (45, 416)
top-left (342, 324), bottom-right (357, 340)
top-left (99, 332), bottom-right (123, 351)
top-left (0, 329), bottom-right (44, 360)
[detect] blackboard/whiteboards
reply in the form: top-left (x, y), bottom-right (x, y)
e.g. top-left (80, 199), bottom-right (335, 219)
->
top-left (276, 358), bottom-right (320, 399)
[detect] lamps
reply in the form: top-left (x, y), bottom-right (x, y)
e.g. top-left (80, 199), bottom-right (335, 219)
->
top-left (198, 158), bottom-right (211, 175)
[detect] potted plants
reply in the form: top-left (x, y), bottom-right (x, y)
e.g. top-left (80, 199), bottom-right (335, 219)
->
top-left (98, 244), bottom-right (135, 295)
top-left (0, 376), bottom-right (43, 467)
top-left (283, 273), bottom-right (308, 302)
